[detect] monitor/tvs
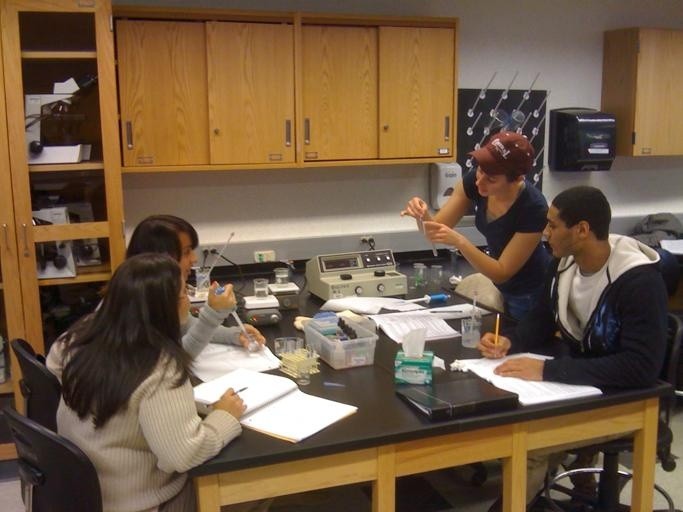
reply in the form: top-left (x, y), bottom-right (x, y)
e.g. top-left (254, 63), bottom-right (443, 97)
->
top-left (364, 312), bottom-right (461, 343)
top-left (394, 376), bottom-right (518, 423)
top-left (450, 353), bottom-right (603, 409)
top-left (189, 343), bottom-right (285, 384)
top-left (193, 367), bottom-right (357, 442)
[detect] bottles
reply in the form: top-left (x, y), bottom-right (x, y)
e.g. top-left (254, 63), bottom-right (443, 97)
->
top-left (469, 132), bottom-right (534, 175)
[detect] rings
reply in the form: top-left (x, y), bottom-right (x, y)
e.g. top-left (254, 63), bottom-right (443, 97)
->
top-left (430, 311), bottom-right (462, 313)
top-left (204, 386), bottom-right (251, 409)
top-left (494, 313), bottom-right (501, 347)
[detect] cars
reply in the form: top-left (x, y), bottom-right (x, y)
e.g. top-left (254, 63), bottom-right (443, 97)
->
top-left (408, 264), bottom-right (426, 290)
top-left (271, 338), bottom-right (302, 356)
top-left (272, 266), bottom-right (289, 289)
top-left (296, 364), bottom-right (310, 386)
top-left (194, 268), bottom-right (210, 293)
top-left (459, 308), bottom-right (482, 349)
top-left (252, 278), bottom-right (271, 300)
top-left (245, 333), bottom-right (262, 358)
top-left (429, 265), bottom-right (442, 291)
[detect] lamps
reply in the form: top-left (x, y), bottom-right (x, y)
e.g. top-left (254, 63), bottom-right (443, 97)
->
top-left (4, 404), bottom-right (103, 512)
top-left (11, 339), bottom-right (61, 432)
top-left (596, 312), bottom-right (682, 510)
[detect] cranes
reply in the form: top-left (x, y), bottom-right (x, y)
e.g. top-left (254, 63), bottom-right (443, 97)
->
top-left (394, 377), bottom-right (518, 424)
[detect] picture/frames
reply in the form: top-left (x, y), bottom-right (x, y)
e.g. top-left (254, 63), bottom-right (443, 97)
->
top-left (190, 278), bottom-right (672, 512)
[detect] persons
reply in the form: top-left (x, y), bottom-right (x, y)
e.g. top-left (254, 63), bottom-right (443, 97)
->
top-left (476, 185), bottom-right (669, 511)
top-left (45, 214), bottom-right (265, 364)
top-left (399, 130), bottom-right (551, 324)
top-left (46, 255), bottom-right (246, 512)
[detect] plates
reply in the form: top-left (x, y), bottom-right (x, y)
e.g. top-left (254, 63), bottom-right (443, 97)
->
top-left (298, 12), bottom-right (460, 168)
top-left (112, 6), bottom-right (298, 172)
top-left (0, 1), bottom-right (126, 460)
top-left (601, 26), bottom-right (683, 156)
